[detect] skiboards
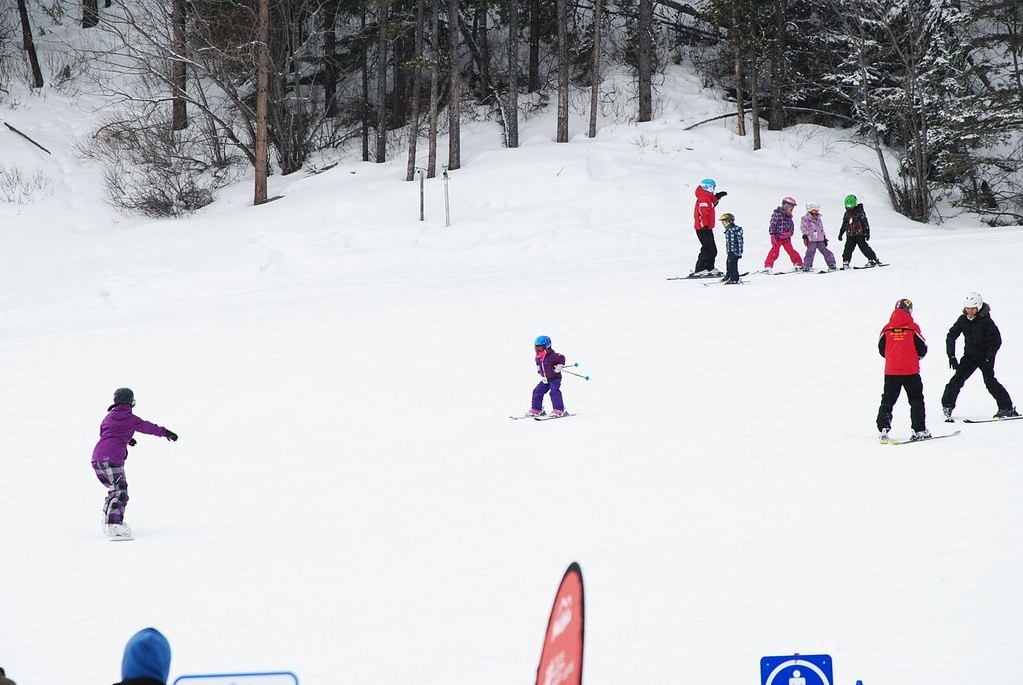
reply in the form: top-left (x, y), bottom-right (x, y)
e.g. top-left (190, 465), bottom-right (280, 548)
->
top-left (509, 410), bottom-right (575, 422)
top-left (879, 429), bottom-right (960, 444)
top-left (665, 270), bottom-right (749, 285)
top-left (940, 415), bottom-right (1022, 422)
top-left (756, 261), bottom-right (889, 275)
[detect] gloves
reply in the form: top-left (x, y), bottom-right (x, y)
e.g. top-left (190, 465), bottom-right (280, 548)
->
top-left (703, 226), bottom-right (711, 239)
top-left (165, 429), bottom-right (178, 442)
top-left (129, 438), bottom-right (136, 447)
top-left (772, 235), bottom-right (777, 246)
top-left (804, 238), bottom-right (808, 246)
top-left (554, 365), bottom-right (563, 373)
top-left (949, 356), bottom-right (959, 370)
top-left (715, 191), bottom-right (727, 200)
top-left (838, 235), bottom-right (843, 241)
top-left (865, 232), bottom-right (870, 240)
top-left (981, 355), bottom-right (994, 370)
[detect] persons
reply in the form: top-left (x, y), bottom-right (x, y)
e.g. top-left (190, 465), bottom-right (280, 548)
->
top-left (693, 178), bottom-right (728, 277)
top-left (114, 628), bottom-right (172, 685)
top-left (840, 194), bottom-right (881, 268)
top-left (942, 291), bottom-right (1019, 420)
top-left (91, 388), bottom-right (178, 535)
top-left (718, 212), bottom-right (745, 283)
top-left (527, 335), bottom-right (568, 416)
top-left (876, 300), bottom-right (934, 441)
top-left (801, 202), bottom-right (838, 272)
top-left (764, 198), bottom-right (804, 272)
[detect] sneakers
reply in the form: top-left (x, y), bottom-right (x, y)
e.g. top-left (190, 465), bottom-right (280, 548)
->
top-left (105, 524), bottom-right (132, 539)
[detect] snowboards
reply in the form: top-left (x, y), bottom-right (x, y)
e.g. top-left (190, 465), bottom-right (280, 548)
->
top-left (103, 520), bottom-right (135, 542)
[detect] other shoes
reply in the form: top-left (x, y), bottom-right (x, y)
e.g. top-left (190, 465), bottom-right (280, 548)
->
top-left (879, 425), bottom-right (931, 439)
top-left (763, 258), bottom-right (880, 273)
top-left (689, 266), bottom-right (741, 285)
top-left (527, 408), bottom-right (565, 416)
top-left (941, 405), bottom-right (1017, 421)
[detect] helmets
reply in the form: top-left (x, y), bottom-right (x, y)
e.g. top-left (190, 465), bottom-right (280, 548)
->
top-left (965, 292), bottom-right (983, 312)
top-left (806, 201), bottom-right (819, 211)
top-left (719, 213), bottom-right (734, 227)
top-left (845, 194), bottom-right (856, 208)
top-left (701, 179), bottom-right (715, 193)
top-left (535, 335), bottom-right (552, 348)
top-left (895, 298), bottom-right (913, 313)
top-left (782, 197), bottom-right (797, 206)
